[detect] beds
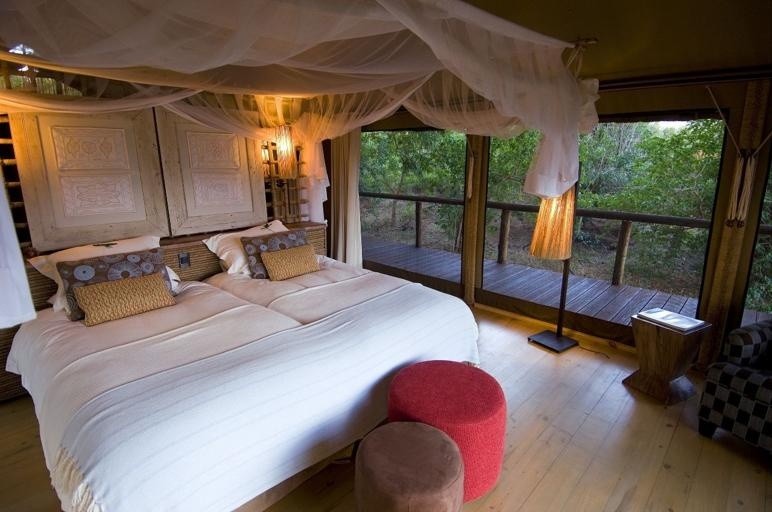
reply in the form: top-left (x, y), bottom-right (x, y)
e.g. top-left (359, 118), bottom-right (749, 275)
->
top-left (4, 251), bottom-right (480, 512)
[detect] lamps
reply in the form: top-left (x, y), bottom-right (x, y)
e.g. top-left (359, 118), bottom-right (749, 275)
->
top-left (526, 160), bottom-right (580, 354)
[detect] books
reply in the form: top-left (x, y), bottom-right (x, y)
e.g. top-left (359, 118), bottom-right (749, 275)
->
top-left (637, 308), bottom-right (707, 333)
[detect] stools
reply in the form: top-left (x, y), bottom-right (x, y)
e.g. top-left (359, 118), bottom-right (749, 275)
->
top-left (353, 359), bottom-right (508, 512)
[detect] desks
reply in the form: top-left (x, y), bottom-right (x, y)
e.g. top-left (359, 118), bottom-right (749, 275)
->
top-left (620, 306), bottom-right (713, 406)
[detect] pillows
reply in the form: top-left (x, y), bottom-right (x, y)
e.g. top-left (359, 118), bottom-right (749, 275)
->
top-left (25, 215), bottom-right (321, 328)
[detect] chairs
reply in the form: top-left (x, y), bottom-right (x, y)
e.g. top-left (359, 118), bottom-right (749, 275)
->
top-left (694, 316), bottom-right (771, 470)
